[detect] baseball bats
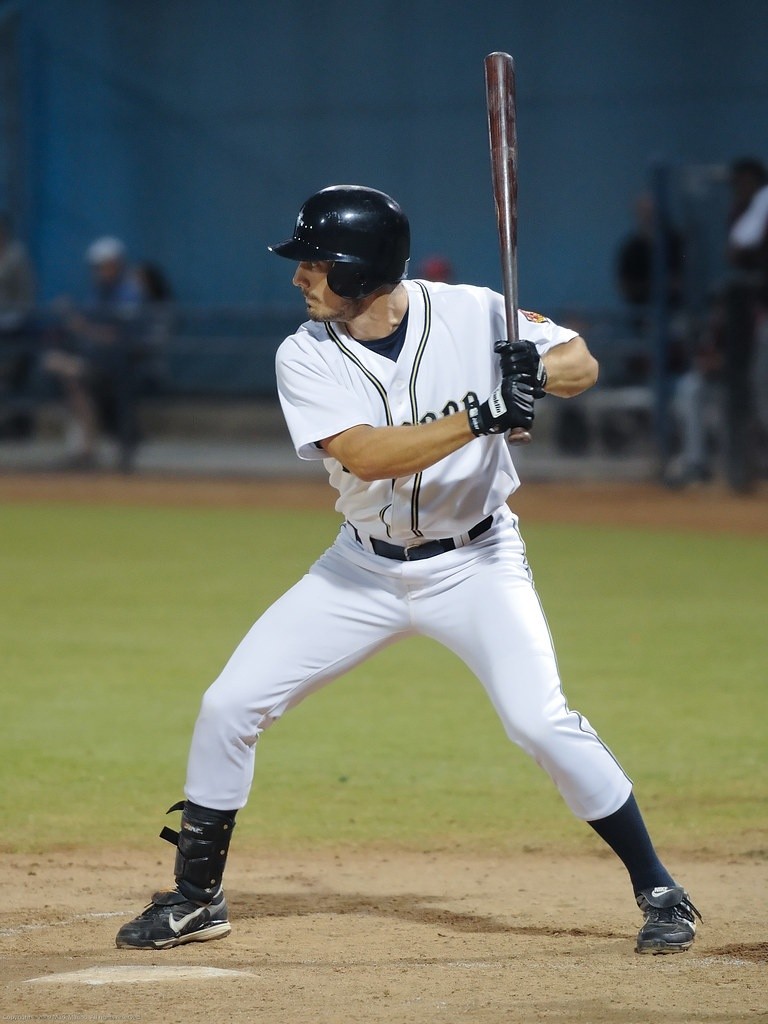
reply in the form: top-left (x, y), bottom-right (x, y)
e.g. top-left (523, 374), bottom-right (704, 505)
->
top-left (480, 50), bottom-right (536, 447)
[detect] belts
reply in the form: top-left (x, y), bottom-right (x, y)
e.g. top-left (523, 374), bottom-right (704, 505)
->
top-left (346, 515), bottom-right (493, 562)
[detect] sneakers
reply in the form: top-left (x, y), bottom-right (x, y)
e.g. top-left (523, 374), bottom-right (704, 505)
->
top-left (116, 891), bottom-right (233, 950)
top-left (635, 886), bottom-right (704, 952)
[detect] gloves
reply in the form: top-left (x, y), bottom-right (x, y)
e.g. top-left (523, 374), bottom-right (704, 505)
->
top-left (467, 373), bottom-right (546, 437)
top-left (494, 340), bottom-right (548, 390)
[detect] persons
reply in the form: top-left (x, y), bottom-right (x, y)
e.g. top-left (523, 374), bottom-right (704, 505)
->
top-left (114, 185), bottom-right (700, 952)
top-left (0, 160), bottom-right (768, 493)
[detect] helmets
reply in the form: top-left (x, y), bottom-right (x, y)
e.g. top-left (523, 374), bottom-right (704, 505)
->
top-left (267, 184), bottom-right (411, 300)
